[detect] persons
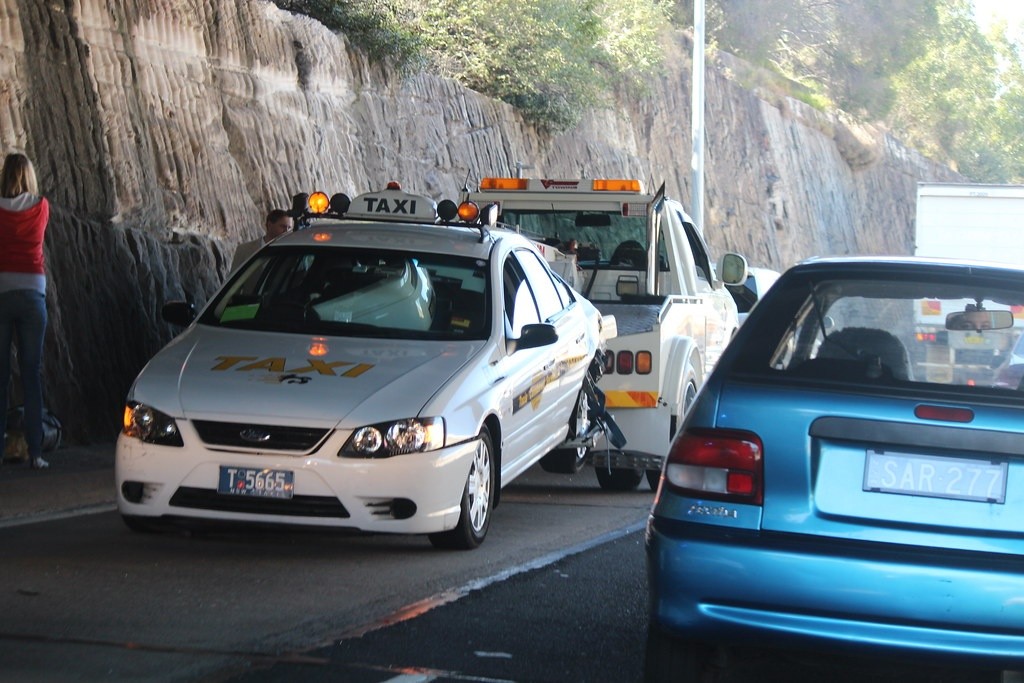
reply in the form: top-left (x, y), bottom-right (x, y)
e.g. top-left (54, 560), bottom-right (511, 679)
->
top-left (0, 152), bottom-right (54, 474)
top-left (949, 312), bottom-right (994, 332)
top-left (230, 209), bottom-right (293, 299)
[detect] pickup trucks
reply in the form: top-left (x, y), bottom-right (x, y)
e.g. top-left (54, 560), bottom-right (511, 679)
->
top-left (458, 178), bottom-right (749, 490)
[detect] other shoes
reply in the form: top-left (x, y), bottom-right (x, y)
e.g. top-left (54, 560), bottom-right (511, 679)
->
top-left (31, 456), bottom-right (48, 471)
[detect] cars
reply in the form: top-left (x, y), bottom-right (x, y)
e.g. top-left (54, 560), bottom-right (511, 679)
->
top-left (115, 178), bottom-right (606, 547)
top-left (645, 256), bottom-right (1024, 683)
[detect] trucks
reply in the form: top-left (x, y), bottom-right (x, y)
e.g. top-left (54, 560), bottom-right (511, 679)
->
top-left (914, 181), bottom-right (1023, 389)
